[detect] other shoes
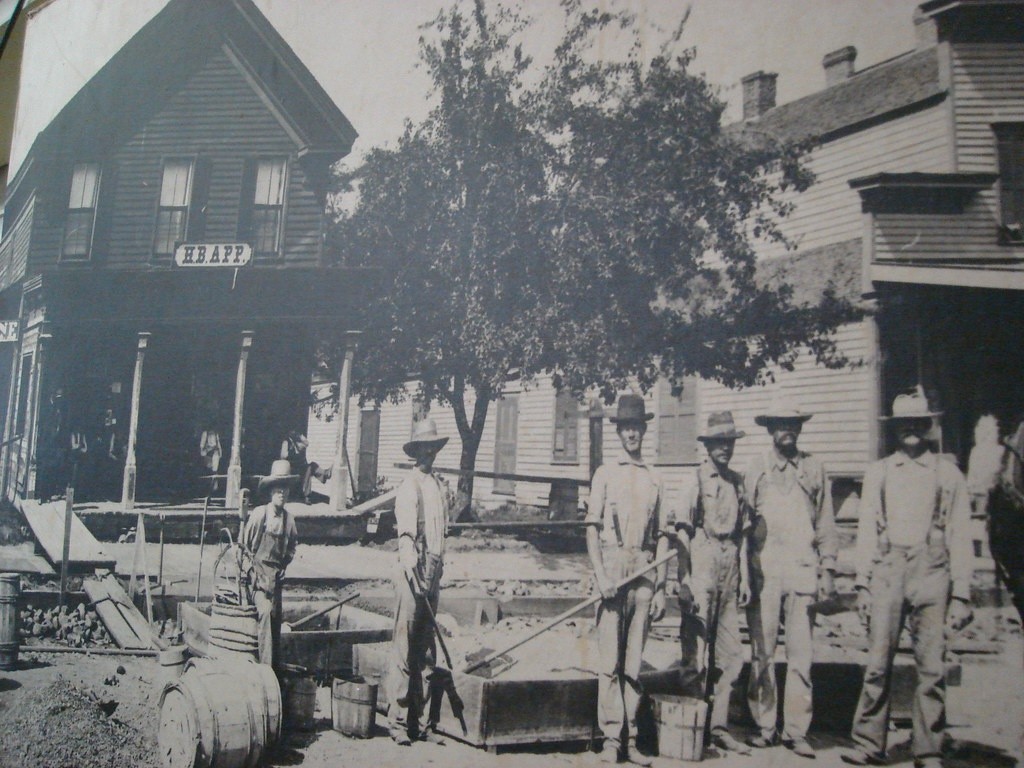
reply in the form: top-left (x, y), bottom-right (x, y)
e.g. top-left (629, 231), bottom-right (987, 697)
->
top-left (425, 728), bottom-right (445, 744)
top-left (750, 735), bottom-right (767, 749)
top-left (599, 746), bottom-right (618, 764)
top-left (393, 732), bottom-right (411, 745)
top-left (705, 731), bottom-right (752, 754)
top-left (793, 736), bottom-right (817, 757)
top-left (626, 746), bottom-right (653, 766)
top-left (839, 748), bottom-right (869, 766)
top-left (914, 755), bottom-right (945, 768)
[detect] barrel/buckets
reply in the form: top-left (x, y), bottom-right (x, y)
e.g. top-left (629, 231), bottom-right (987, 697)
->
top-left (157, 674), bottom-right (259, 768)
top-left (180, 657), bottom-right (282, 748)
top-left (548, 483), bottom-right (579, 522)
top-left (276, 672), bottom-right (316, 726)
top-left (651, 694), bottom-right (708, 762)
top-left (0, 573), bottom-right (20, 669)
top-left (333, 675), bottom-right (378, 739)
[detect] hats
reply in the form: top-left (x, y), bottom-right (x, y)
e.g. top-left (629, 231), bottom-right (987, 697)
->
top-left (258, 460), bottom-right (300, 493)
top-left (696, 410), bottom-right (746, 441)
top-left (754, 400), bottom-right (813, 426)
top-left (403, 421), bottom-right (449, 458)
top-left (876, 392), bottom-right (944, 421)
top-left (609, 394), bottom-right (655, 422)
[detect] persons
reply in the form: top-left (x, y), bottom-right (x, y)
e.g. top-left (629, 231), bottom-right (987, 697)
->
top-left (839, 388), bottom-right (975, 768)
top-left (243, 459), bottom-right (300, 671)
top-left (581, 391), bottom-right (838, 766)
top-left (386, 420), bottom-right (450, 747)
top-left (279, 426), bottom-right (333, 506)
top-left (199, 427), bottom-right (224, 492)
